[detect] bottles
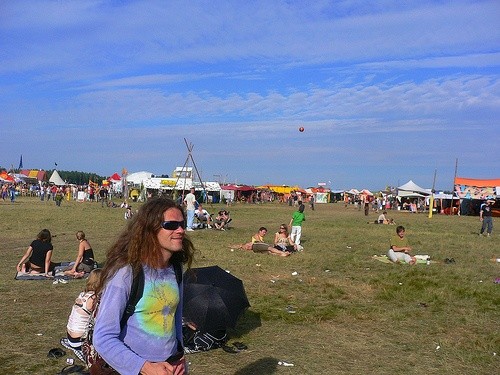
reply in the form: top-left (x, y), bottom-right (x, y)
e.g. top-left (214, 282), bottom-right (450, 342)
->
top-left (426, 258), bottom-right (430, 265)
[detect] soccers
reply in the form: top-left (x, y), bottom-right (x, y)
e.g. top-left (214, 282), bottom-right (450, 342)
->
top-left (299, 127), bottom-right (304, 132)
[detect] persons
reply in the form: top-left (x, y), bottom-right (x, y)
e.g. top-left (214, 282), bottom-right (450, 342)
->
top-left (184, 186), bottom-right (232, 233)
top-left (17, 228), bottom-right (55, 278)
top-left (252, 227), bottom-right (290, 257)
top-left (366, 211), bottom-right (396, 226)
top-left (479, 198), bottom-right (495, 237)
top-left (2, 184), bottom-right (107, 208)
top-left (183, 320), bottom-right (230, 350)
top-left (343, 193), bottom-right (425, 215)
top-left (64, 231), bottom-right (94, 279)
top-left (263, 191), bottom-right (314, 209)
top-left (275, 204), bottom-right (307, 252)
top-left (67, 195), bottom-right (202, 375)
top-left (389, 226), bottom-right (417, 266)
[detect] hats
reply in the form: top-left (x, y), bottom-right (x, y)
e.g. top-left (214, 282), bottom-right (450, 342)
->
top-left (218, 208), bottom-right (229, 215)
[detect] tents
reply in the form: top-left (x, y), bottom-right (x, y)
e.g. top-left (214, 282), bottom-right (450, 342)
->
top-left (348, 188), bottom-right (374, 196)
top-left (221, 184), bottom-right (331, 196)
top-left (0, 169), bottom-right (67, 186)
top-left (107, 171), bottom-right (121, 183)
top-left (397, 177), bottom-right (500, 202)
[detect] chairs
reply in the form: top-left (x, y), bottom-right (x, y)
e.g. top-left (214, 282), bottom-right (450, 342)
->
top-left (218, 210), bottom-right (232, 229)
top-left (196, 215), bottom-right (212, 230)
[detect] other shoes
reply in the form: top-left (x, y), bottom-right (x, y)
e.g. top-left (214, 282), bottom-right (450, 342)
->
top-left (199, 224), bottom-right (203, 228)
top-left (187, 227), bottom-right (194, 231)
top-left (208, 225), bottom-right (212, 229)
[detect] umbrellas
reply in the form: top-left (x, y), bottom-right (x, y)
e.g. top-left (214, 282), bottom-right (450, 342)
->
top-left (182, 264), bottom-right (251, 332)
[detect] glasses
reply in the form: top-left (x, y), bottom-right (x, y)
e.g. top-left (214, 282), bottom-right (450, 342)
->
top-left (160, 217), bottom-right (187, 230)
top-left (280, 227), bottom-right (284, 229)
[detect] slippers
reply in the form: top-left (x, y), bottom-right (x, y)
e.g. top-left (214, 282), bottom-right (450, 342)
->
top-left (53, 278), bottom-right (58, 284)
top-left (59, 364), bottom-right (90, 375)
top-left (444, 258), bottom-right (456, 264)
top-left (48, 348), bottom-right (64, 360)
top-left (58, 278), bottom-right (68, 284)
top-left (232, 341), bottom-right (248, 349)
top-left (222, 345), bottom-right (237, 354)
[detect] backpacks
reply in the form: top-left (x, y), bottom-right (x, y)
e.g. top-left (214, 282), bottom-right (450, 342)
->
top-left (88, 260), bottom-right (184, 375)
